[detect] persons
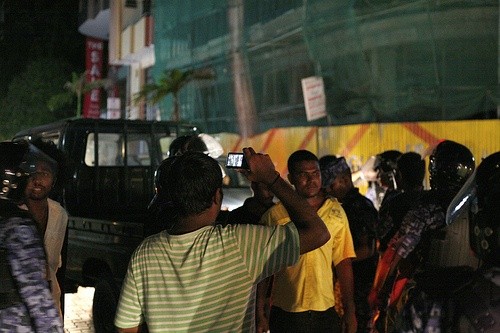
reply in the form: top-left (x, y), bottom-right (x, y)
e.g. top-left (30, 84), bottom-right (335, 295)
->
top-left (221, 138), bottom-right (500, 333)
top-left (141, 135), bottom-right (232, 237)
top-left (0, 138), bottom-right (74, 333)
top-left (113, 148), bottom-right (330, 333)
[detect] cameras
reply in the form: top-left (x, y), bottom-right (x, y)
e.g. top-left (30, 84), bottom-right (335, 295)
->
top-left (225, 152), bottom-right (250, 170)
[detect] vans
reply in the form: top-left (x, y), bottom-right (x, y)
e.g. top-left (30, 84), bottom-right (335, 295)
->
top-left (4, 119), bottom-right (196, 222)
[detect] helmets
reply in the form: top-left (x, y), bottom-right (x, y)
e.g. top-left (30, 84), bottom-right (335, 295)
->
top-left (373, 150), bottom-right (403, 187)
top-left (429, 140), bottom-right (475, 190)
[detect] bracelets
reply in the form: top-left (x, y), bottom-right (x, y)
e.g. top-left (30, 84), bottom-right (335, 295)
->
top-left (267, 175), bottom-right (280, 191)
